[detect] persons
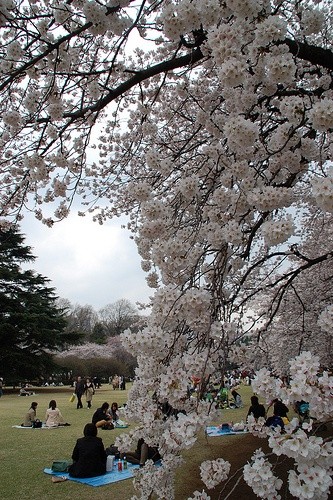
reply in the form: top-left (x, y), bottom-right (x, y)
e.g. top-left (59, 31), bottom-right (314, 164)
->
top-left (46, 400), bottom-right (70, 426)
top-left (92, 401), bottom-right (112, 427)
top-left (273, 398), bottom-right (289, 418)
top-left (84, 377), bottom-right (94, 409)
top-left (75, 376), bottom-right (85, 409)
top-left (112, 374), bottom-right (126, 390)
top-left (293, 401), bottom-right (308, 418)
top-left (116, 439), bottom-right (163, 470)
top-left (68, 423), bottom-right (108, 479)
top-left (245, 396), bottom-right (266, 425)
top-left (93, 376), bottom-right (103, 389)
top-left (45, 382), bottom-right (64, 387)
top-left (230, 390), bottom-right (243, 408)
top-left (228, 368), bottom-right (256, 386)
top-left (0, 377), bottom-right (5, 397)
top-left (20, 387), bottom-right (29, 396)
top-left (24, 402), bottom-right (38, 427)
top-left (204, 380), bottom-right (231, 409)
top-left (130, 375), bottom-right (133, 385)
top-left (109, 402), bottom-right (119, 421)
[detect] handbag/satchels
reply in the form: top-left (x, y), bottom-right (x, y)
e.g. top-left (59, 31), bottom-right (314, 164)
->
top-left (106, 447), bottom-right (120, 459)
top-left (32, 419), bottom-right (42, 428)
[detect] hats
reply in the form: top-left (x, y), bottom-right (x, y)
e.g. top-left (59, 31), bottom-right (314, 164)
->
top-left (273, 402), bottom-right (289, 415)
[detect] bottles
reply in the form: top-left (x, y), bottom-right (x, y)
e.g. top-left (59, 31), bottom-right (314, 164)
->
top-left (118, 459), bottom-right (122, 471)
top-left (124, 456), bottom-right (127, 468)
